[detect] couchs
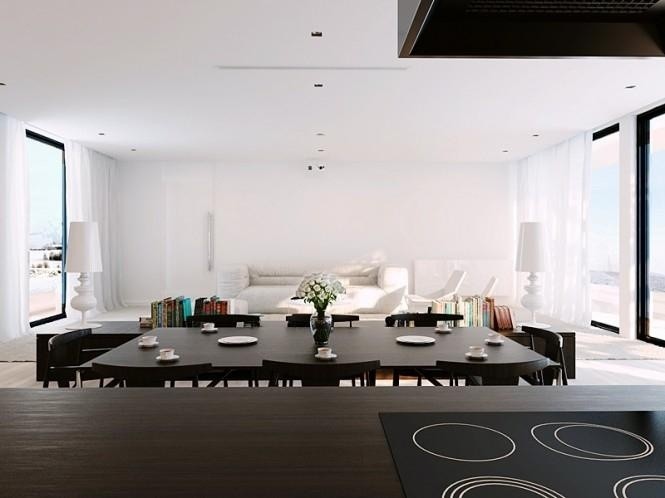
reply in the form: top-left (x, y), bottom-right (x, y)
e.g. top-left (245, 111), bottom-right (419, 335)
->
top-left (214, 264), bottom-right (409, 313)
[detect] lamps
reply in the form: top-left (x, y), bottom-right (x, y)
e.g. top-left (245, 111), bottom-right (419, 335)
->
top-left (61, 220), bottom-right (103, 331)
top-left (513, 219), bottom-right (553, 331)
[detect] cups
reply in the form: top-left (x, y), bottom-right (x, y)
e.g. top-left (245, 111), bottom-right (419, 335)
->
top-left (488, 334), bottom-right (501, 341)
top-left (317, 348), bottom-right (331, 356)
top-left (439, 324), bottom-right (448, 329)
top-left (159, 349), bottom-right (172, 359)
top-left (204, 323), bottom-right (214, 330)
top-left (142, 336), bottom-right (156, 344)
top-left (469, 346), bottom-right (484, 357)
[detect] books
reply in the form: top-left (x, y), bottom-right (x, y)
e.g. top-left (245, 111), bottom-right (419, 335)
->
top-left (430, 295), bottom-right (514, 331)
top-left (139, 296), bottom-right (249, 329)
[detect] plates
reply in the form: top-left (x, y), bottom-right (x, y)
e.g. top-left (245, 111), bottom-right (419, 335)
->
top-left (217, 336), bottom-right (258, 345)
top-left (397, 335), bottom-right (436, 344)
top-left (139, 341), bottom-right (159, 348)
top-left (434, 328), bottom-right (452, 332)
top-left (485, 339), bottom-right (504, 344)
top-left (201, 328), bottom-right (217, 332)
top-left (315, 354), bottom-right (337, 360)
top-left (466, 352), bottom-right (489, 359)
top-left (156, 355), bottom-right (180, 360)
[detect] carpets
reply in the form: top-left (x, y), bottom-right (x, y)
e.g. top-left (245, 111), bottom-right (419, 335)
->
top-left (0, 321), bottom-right (665, 366)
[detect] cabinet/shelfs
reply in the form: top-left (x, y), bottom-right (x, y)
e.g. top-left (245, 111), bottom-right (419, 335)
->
top-left (36, 313), bottom-right (221, 387)
top-left (426, 308), bottom-right (576, 378)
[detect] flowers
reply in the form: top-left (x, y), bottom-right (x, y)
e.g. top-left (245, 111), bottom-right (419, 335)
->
top-left (291, 274), bottom-right (348, 317)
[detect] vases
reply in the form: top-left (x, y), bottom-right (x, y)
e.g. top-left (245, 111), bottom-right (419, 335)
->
top-left (310, 310), bottom-right (332, 345)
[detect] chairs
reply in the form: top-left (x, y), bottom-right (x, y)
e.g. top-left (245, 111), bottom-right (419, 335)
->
top-left (480, 274), bottom-right (499, 302)
top-left (184, 314), bottom-right (263, 389)
top-left (382, 314), bottom-right (467, 387)
top-left (283, 314), bottom-right (360, 387)
top-left (48, 330), bottom-right (138, 389)
top-left (405, 269), bottom-right (465, 309)
top-left (517, 325), bottom-right (566, 386)
